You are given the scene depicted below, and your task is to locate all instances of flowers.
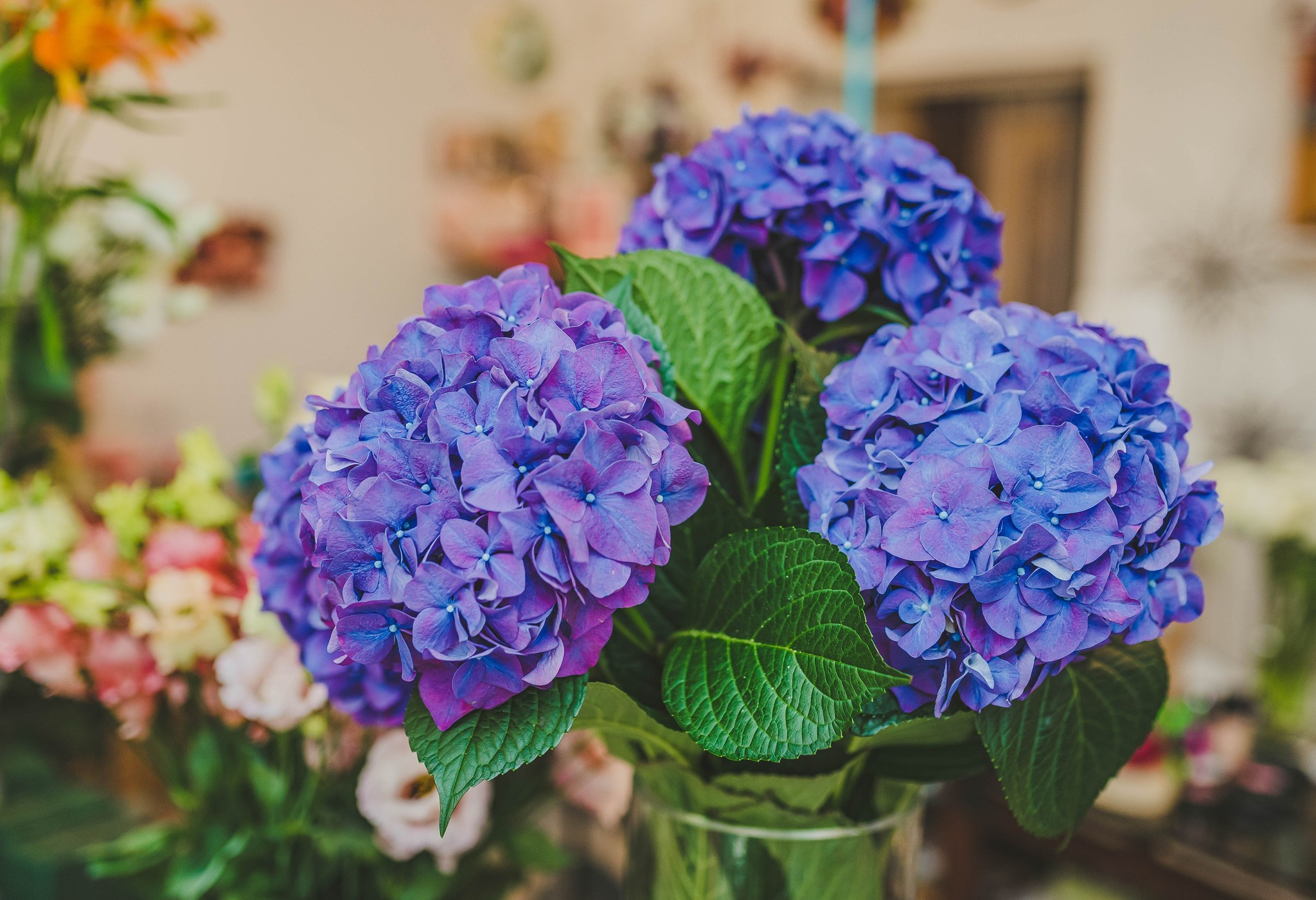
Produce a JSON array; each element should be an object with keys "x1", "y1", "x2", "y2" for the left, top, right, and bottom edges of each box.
[{"x1": 0, "y1": 0, "x2": 1265, "y2": 900}]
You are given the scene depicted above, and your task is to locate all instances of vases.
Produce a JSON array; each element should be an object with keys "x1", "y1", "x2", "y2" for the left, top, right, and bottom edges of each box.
[{"x1": 618, "y1": 759, "x2": 927, "y2": 900}]
